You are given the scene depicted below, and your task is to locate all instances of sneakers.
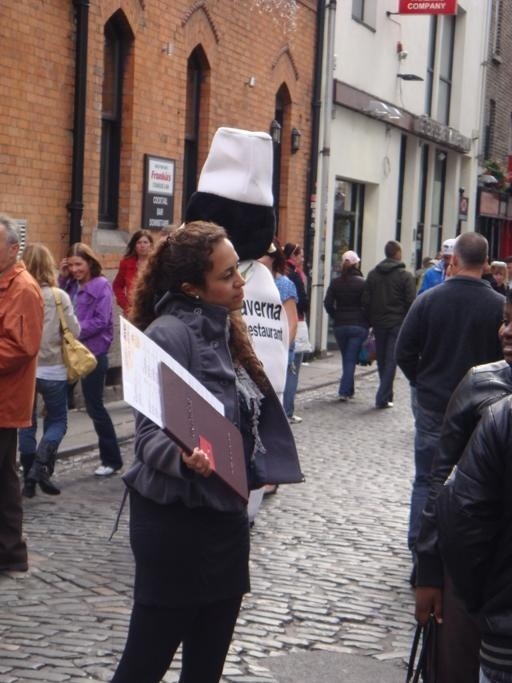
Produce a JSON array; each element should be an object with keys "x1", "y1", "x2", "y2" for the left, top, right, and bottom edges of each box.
[
  {"x1": 288, "y1": 414, "x2": 303, "y2": 423},
  {"x1": 377, "y1": 402, "x2": 394, "y2": 408},
  {"x1": 93, "y1": 463, "x2": 117, "y2": 476},
  {"x1": 340, "y1": 391, "x2": 358, "y2": 401}
]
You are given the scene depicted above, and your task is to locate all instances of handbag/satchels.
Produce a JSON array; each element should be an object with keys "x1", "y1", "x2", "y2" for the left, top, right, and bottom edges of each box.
[
  {"x1": 293, "y1": 320, "x2": 312, "y2": 353},
  {"x1": 62, "y1": 333, "x2": 97, "y2": 386},
  {"x1": 358, "y1": 328, "x2": 377, "y2": 366}
]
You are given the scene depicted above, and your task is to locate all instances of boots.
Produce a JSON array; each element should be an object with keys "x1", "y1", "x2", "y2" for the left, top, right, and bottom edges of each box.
[{"x1": 20, "y1": 441, "x2": 60, "y2": 496}]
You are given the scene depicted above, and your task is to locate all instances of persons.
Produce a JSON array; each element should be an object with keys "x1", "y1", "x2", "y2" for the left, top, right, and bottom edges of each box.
[
  {"x1": 2, "y1": 219, "x2": 43, "y2": 570},
  {"x1": 366, "y1": 241, "x2": 416, "y2": 409},
  {"x1": 110, "y1": 220, "x2": 306, "y2": 682},
  {"x1": 113, "y1": 230, "x2": 153, "y2": 316},
  {"x1": 260, "y1": 236, "x2": 308, "y2": 495},
  {"x1": 323, "y1": 250, "x2": 369, "y2": 402},
  {"x1": 394, "y1": 230, "x2": 512, "y2": 682},
  {"x1": 21, "y1": 241, "x2": 124, "y2": 496}
]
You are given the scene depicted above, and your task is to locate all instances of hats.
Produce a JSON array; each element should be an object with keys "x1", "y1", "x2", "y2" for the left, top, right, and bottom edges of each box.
[
  {"x1": 441, "y1": 238, "x2": 457, "y2": 256},
  {"x1": 342, "y1": 250, "x2": 360, "y2": 265}
]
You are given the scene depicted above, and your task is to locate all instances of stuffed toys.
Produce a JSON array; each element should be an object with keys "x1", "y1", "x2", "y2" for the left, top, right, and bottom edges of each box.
[{"x1": 186, "y1": 126, "x2": 288, "y2": 530}]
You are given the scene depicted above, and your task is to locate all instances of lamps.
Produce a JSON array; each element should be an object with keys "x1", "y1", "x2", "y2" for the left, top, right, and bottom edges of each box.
[
  {"x1": 245, "y1": 76, "x2": 256, "y2": 87},
  {"x1": 291, "y1": 128, "x2": 301, "y2": 153},
  {"x1": 162, "y1": 41, "x2": 174, "y2": 55},
  {"x1": 271, "y1": 119, "x2": 282, "y2": 144}
]
